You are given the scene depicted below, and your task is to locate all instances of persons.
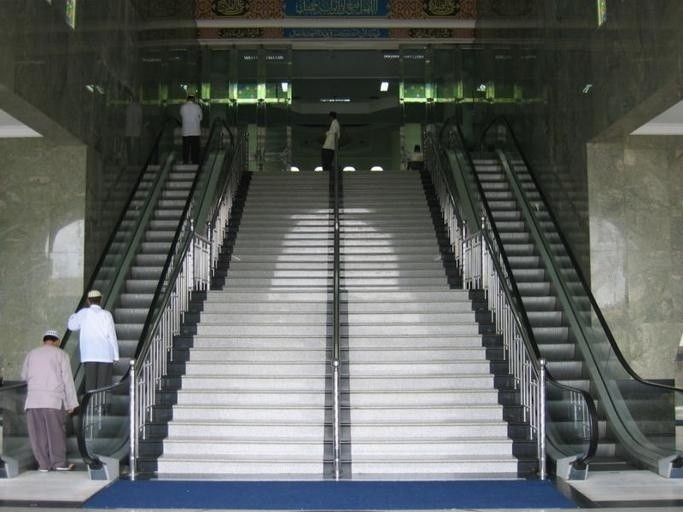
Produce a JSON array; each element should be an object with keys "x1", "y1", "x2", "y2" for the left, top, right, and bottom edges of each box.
[
  {"x1": 67, "y1": 289, "x2": 119, "y2": 406},
  {"x1": 123, "y1": 96, "x2": 144, "y2": 166},
  {"x1": 320, "y1": 111, "x2": 341, "y2": 171},
  {"x1": 178, "y1": 95, "x2": 203, "y2": 163},
  {"x1": 20, "y1": 330, "x2": 79, "y2": 472}
]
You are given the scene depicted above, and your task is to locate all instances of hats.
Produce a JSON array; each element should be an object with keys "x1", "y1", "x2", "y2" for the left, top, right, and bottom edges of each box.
[
  {"x1": 87, "y1": 289, "x2": 103, "y2": 298},
  {"x1": 41, "y1": 329, "x2": 61, "y2": 343}
]
